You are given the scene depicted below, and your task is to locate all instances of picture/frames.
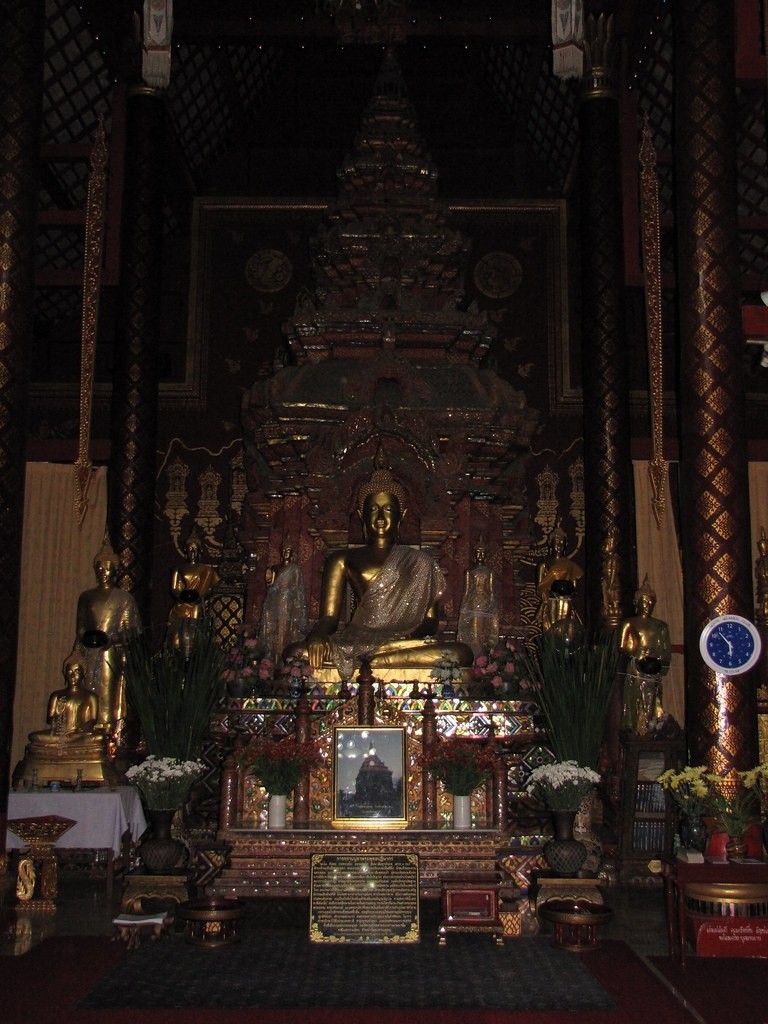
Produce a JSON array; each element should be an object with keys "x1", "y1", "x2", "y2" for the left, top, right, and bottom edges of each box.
[{"x1": 332, "y1": 724, "x2": 410, "y2": 823}]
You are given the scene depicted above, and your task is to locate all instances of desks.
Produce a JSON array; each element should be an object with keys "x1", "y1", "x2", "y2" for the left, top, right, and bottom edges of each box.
[{"x1": 7, "y1": 786, "x2": 149, "y2": 898}]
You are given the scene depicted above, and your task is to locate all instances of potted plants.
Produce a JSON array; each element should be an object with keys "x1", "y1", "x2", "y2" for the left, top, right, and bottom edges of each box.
[
  {"x1": 104, "y1": 608, "x2": 256, "y2": 847},
  {"x1": 516, "y1": 621, "x2": 624, "y2": 834}
]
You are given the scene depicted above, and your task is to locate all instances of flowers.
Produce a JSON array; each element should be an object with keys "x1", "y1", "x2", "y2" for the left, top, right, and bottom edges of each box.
[
  {"x1": 235, "y1": 733, "x2": 327, "y2": 796},
  {"x1": 430, "y1": 649, "x2": 464, "y2": 686},
  {"x1": 748, "y1": 753, "x2": 768, "y2": 826},
  {"x1": 213, "y1": 622, "x2": 270, "y2": 687},
  {"x1": 705, "y1": 768, "x2": 758, "y2": 837},
  {"x1": 656, "y1": 765, "x2": 724, "y2": 823},
  {"x1": 468, "y1": 636, "x2": 545, "y2": 692},
  {"x1": 522, "y1": 757, "x2": 603, "y2": 810},
  {"x1": 275, "y1": 646, "x2": 316, "y2": 676},
  {"x1": 123, "y1": 755, "x2": 208, "y2": 812},
  {"x1": 415, "y1": 735, "x2": 501, "y2": 796}
]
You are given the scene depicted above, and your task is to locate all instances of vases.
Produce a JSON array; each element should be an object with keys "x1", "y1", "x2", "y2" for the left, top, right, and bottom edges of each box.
[
  {"x1": 681, "y1": 816, "x2": 707, "y2": 854},
  {"x1": 705, "y1": 817, "x2": 732, "y2": 860},
  {"x1": 738, "y1": 817, "x2": 763, "y2": 858},
  {"x1": 288, "y1": 676, "x2": 307, "y2": 700},
  {"x1": 488, "y1": 677, "x2": 522, "y2": 703},
  {"x1": 441, "y1": 680, "x2": 456, "y2": 698},
  {"x1": 452, "y1": 794, "x2": 472, "y2": 832},
  {"x1": 268, "y1": 794, "x2": 286, "y2": 832},
  {"x1": 552, "y1": 808, "x2": 577, "y2": 842},
  {"x1": 140, "y1": 810, "x2": 186, "y2": 873},
  {"x1": 225, "y1": 669, "x2": 253, "y2": 699}
]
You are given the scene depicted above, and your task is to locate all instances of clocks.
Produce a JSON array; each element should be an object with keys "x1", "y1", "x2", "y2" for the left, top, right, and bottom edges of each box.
[{"x1": 699, "y1": 614, "x2": 761, "y2": 675}]
